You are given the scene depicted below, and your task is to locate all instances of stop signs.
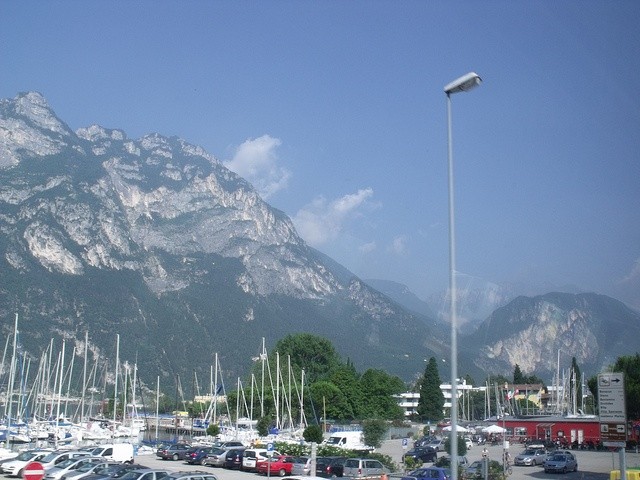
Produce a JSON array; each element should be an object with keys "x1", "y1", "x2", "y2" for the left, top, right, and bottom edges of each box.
[{"x1": 22, "y1": 461, "x2": 44, "y2": 480}]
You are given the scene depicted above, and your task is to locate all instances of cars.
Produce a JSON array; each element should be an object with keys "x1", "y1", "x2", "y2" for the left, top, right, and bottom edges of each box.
[
  {"x1": 514, "y1": 445, "x2": 547, "y2": 466},
  {"x1": 1, "y1": 451, "x2": 52, "y2": 478},
  {"x1": 545, "y1": 454, "x2": 576, "y2": 474},
  {"x1": 61, "y1": 461, "x2": 122, "y2": 480},
  {"x1": 77, "y1": 465, "x2": 151, "y2": 480},
  {"x1": 402, "y1": 447, "x2": 437, "y2": 462},
  {"x1": 43, "y1": 456, "x2": 107, "y2": 480},
  {"x1": 426, "y1": 440, "x2": 446, "y2": 452},
  {"x1": 120, "y1": 469, "x2": 172, "y2": 480},
  {"x1": 206, "y1": 448, "x2": 229, "y2": 467},
  {"x1": 256, "y1": 456, "x2": 295, "y2": 476},
  {"x1": 291, "y1": 456, "x2": 323, "y2": 475}
]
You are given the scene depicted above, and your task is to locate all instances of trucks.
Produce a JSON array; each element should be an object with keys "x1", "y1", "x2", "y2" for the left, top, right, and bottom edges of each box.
[
  {"x1": 326, "y1": 432, "x2": 376, "y2": 454},
  {"x1": 92, "y1": 444, "x2": 134, "y2": 465}
]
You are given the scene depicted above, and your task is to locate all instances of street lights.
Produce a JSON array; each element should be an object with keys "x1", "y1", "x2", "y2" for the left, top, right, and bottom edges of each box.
[{"x1": 444, "y1": 71, "x2": 483, "y2": 480}]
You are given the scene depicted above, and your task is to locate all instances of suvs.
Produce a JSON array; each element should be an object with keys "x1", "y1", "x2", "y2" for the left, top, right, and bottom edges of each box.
[
  {"x1": 463, "y1": 438, "x2": 473, "y2": 450},
  {"x1": 401, "y1": 467, "x2": 450, "y2": 480},
  {"x1": 159, "y1": 470, "x2": 217, "y2": 480},
  {"x1": 157, "y1": 444, "x2": 192, "y2": 461},
  {"x1": 41, "y1": 450, "x2": 92, "y2": 470},
  {"x1": 213, "y1": 441, "x2": 246, "y2": 452},
  {"x1": 316, "y1": 457, "x2": 348, "y2": 478},
  {"x1": 185, "y1": 446, "x2": 212, "y2": 465},
  {"x1": 226, "y1": 449, "x2": 242, "y2": 469}
]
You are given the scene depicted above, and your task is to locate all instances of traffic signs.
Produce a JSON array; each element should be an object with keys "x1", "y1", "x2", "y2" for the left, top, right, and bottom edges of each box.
[
  {"x1": 596, "y1": 373, "x2": 626, "y2": 441},
  {"x1": 267, "y1": 443, "x2": 274, "y2": 451}
]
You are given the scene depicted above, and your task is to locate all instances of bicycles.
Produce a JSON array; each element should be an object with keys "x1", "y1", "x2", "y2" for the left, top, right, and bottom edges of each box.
[{"x1": 506, "y1": 462, "x2": 513, "y2": 474}]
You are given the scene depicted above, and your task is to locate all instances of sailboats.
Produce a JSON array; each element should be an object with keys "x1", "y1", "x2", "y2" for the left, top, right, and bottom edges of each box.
[
  {"x1": 0, "y1": 311, "x2": 148, "y2": 443},
  {"x1": 169, "y1": 336, "x2": 330, "y2": 441}
]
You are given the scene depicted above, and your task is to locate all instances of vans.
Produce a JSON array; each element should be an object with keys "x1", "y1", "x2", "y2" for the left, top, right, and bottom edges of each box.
[
  {"x1": 242, "y1": 448, "x2": 282, "y2": 472},
  {"x1": 343, "y1": 458, "x2": 390, "y2": 480}
]
